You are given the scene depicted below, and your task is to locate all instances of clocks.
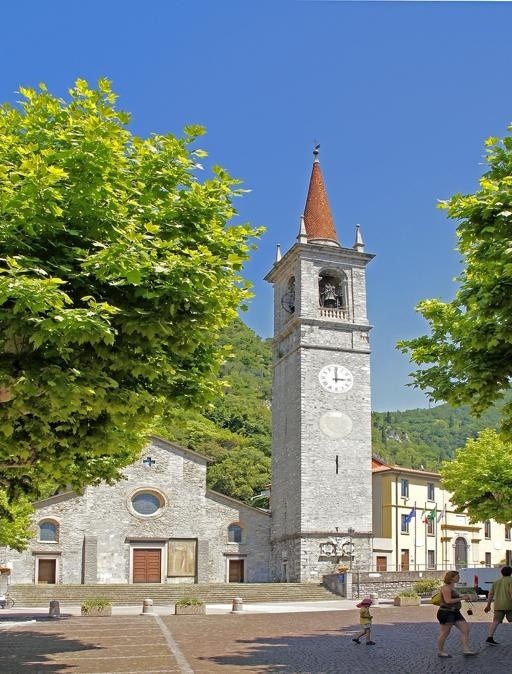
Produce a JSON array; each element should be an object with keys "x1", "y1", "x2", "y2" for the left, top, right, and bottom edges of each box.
[{"x1": 317, "y1": 362, "x2": 354, "y2": 395}]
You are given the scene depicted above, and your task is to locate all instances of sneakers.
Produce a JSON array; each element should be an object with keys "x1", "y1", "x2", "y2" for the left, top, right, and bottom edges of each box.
[
  {"x1": 352, "y1": 638, "x2": 377, "y2": 646},
  {"x1": 437, "y1": 637, "x2": 500, "y2": 658}
]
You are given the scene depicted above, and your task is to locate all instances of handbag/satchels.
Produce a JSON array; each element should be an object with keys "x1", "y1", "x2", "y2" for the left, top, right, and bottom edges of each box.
[{"x1": 430, "y1": 591, "x2": 441, "y2": 606}]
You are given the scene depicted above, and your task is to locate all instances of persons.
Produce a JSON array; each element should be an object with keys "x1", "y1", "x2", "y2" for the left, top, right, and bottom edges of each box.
[
  {"x1": 351, "y1": 598, "x2": 376, "y2": 645},
  {"x1": 437, "y1": 571, "x2": 478, "y2": 657},
  {"x1": 483, "y1": 566, "x2": 511, "y2": 644}
]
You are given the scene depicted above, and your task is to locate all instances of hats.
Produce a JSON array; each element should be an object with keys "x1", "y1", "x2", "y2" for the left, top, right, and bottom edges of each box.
[{"x1": 355, "y1": 598, "x2": 372, "y2": 608}]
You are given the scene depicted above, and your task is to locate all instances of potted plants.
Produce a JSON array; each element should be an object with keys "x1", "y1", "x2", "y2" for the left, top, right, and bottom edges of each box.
[
  {"x1": 394, "y1": 590, "x2": 421, "y2": 605},
  {"x1": 82, "y1": 597, "x2": 112, "y2": 616},
  {"x1": 175, "y1": 597, "x2": 208, "y2": 615}
]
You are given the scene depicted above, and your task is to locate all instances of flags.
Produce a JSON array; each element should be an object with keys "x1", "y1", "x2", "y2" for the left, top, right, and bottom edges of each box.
[
  {"x1": 420, "y1": 508, "x2": 429, "y2": 524},
  {"x1": 404, "y1": 505, "x2": 416, "y2": 523},
  {"x1": 437, "y1": 507, "x2": 445, "y2": 523},
  {"x1": 427, "y1": 507, "x2": 436, "y2": 522}
]
